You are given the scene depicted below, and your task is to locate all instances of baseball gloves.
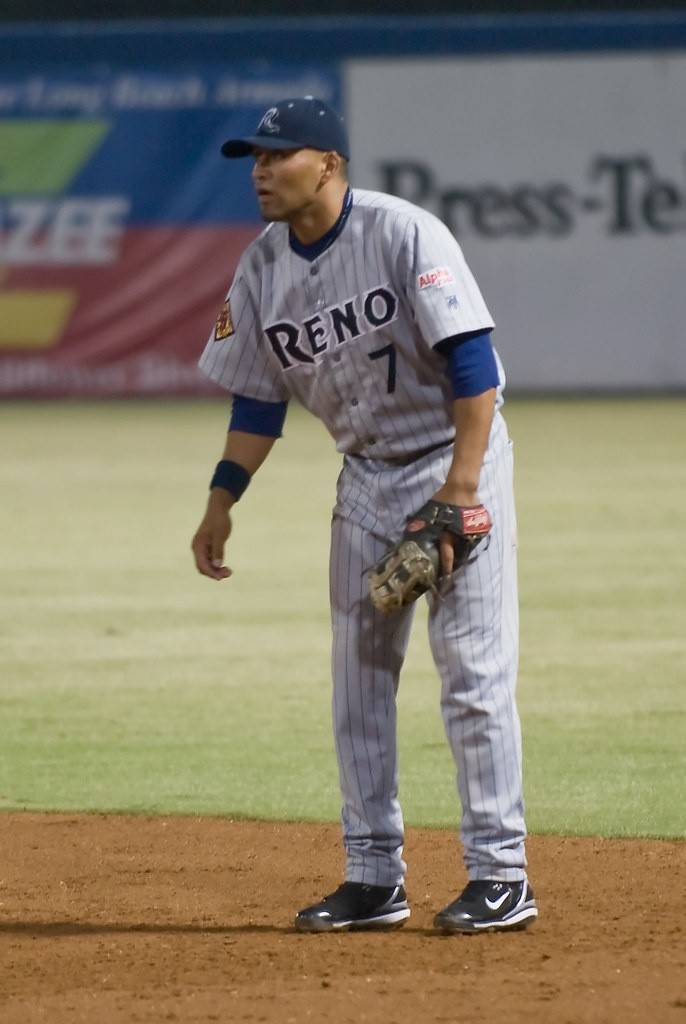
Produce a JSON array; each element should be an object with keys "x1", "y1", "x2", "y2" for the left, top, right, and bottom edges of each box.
[{"x1": 360, "y1": 499, "x2": 494, "y2": 639}]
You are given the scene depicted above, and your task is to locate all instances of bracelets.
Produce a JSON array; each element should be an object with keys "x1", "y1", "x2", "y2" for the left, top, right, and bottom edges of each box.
[{"x1": 209, "y1": 459, "x2": 251, "y2": 501}]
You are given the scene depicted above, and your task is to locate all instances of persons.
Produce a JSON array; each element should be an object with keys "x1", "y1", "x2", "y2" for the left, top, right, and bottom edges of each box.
[{"x1": 191, "y1": 96, "x2": 538, "y2": 934}]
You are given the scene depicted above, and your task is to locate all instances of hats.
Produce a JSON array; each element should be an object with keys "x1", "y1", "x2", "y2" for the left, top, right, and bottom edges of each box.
[{"x1": 221, "y1": 94, "x2": 349, "y2": 160}]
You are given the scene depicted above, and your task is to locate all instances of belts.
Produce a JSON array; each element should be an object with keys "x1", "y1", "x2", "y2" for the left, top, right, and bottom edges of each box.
[{"x1": 382, "y1": 437, "x2": 454, "y2": 467}]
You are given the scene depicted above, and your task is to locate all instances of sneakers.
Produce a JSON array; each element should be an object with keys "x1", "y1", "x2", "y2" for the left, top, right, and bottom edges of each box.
[
  {"x1": 295, "y1": 881, "x2": 410, "y2": 933},
  {"x1": 433, "y1": 880, "x2": 538, "y2": 935}
]
